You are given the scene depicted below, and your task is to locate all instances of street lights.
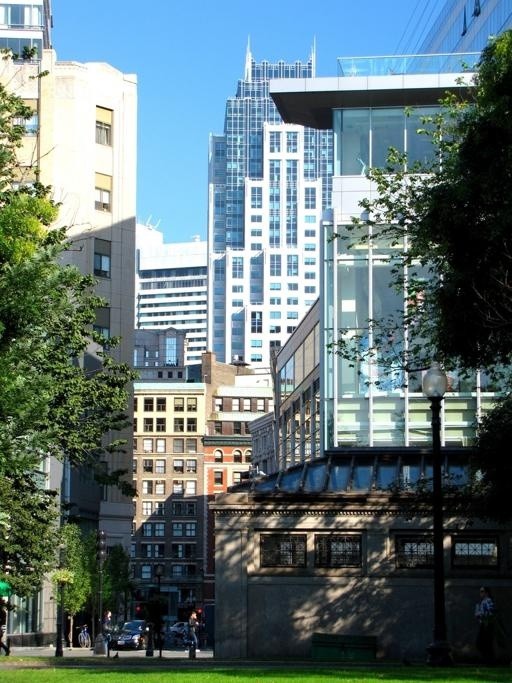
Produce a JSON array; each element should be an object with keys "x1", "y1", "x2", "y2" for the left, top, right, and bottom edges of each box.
[{"x1": 421, "y1": 362, "x2": 448, "y2": 668}]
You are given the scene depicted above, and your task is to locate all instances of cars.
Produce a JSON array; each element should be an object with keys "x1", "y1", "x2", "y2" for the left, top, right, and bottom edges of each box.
[{"x1": 111, "y1": 620, "x2": 189, "y2": 650}]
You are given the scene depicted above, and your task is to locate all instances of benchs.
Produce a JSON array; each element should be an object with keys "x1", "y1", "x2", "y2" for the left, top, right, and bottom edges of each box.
[{"x1": 309, "y1": 632, "x2": 379, "y2": 659}]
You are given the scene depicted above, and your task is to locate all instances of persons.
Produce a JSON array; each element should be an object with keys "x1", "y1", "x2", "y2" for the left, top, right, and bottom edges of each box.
[
  {"x1": 0, "y1": 607, "x2": 11, "y2": 656},
  {"x1": 185, "y1": 611, "x2": 201, "y2": 652},
  {"x1": 102, "y1": 608, "x2": 112, "y2": 650},
  {"x1": 474, "y1": 586, "x2": 497, "y2": 667}
]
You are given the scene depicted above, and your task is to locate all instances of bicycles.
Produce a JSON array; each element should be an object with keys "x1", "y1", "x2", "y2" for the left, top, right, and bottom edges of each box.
[{"x1": 76, "y1": 624, "x2": 91, "y2": 648}]
